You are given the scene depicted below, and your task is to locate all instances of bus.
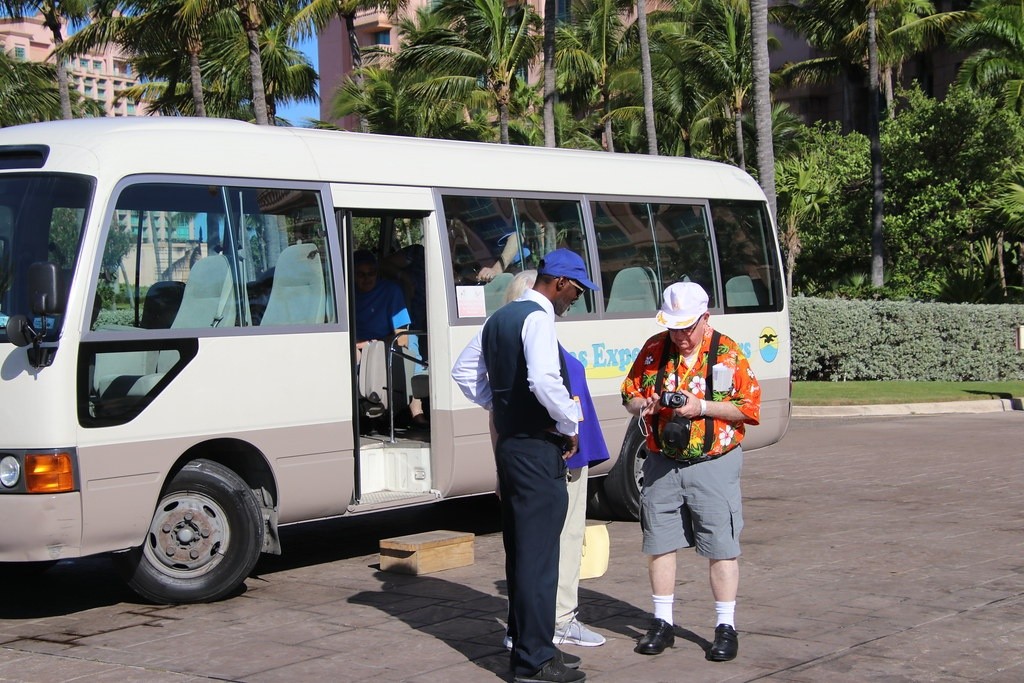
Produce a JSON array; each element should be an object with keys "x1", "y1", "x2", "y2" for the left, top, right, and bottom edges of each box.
[{"x1": 0, "y1": 117, "x2": 789, "y2": 604}]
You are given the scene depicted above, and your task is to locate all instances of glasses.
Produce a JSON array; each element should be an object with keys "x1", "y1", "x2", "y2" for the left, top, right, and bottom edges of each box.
[
  {"x1": 356, "y1": 270, "x2": 377, "y2": 277},
  {"x1": 554, "y1": 276, "x2": 585, "y2": 296},
  {"x1": 667, "y1": 313, "x2": 706, "y2": 334}
]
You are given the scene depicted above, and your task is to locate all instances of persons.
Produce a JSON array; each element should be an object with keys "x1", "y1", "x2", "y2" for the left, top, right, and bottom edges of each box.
[
  {"x1": 451, "y1": 270, "x2": 611, "y2": 647},
  {"x1": 355, "y1": 247, "x2": 411, "y2": 434},
  {"x1": 466, "y1": 199, "x2": 535, "y2": 283},
  {"x1": 620, "y1": 282, "x2": 761, "y2": 661}
]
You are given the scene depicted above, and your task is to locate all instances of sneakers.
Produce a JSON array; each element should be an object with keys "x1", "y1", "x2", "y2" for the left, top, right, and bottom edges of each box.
[
  {"x1": 553, "y1": 611, "x2": 605, "y2": 646},
  {"x1": 516, "y1": 657, "x2": 586, "y2": 683},
  {"x1": 554, "y1": 648, "x2": 582, "y2": 669}
]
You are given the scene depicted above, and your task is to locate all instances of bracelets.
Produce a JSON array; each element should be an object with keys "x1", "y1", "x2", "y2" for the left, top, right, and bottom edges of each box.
[
  {"x1": 368, "y1": 338, "x2": 373, "y2": 344},
  {"x1": 699, "y1": 399, "x2": 706, "y2": 415}
]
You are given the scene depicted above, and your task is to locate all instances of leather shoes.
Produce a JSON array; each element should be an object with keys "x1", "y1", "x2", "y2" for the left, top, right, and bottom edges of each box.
[
  {"x1": 710, "y1": 624, "x2": 738, "y2": 659},
  {"x1": 639, "y1": 617, "x2": 675, "y2": 653}
]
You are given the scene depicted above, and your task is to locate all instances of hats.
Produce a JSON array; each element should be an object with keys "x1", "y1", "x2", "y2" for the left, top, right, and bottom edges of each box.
[
  {"x1": 656, "y1": 281, "x2": 709, "y2": 329},
  {"x1": 538, "y1": 248, "x2": 598, "y2": 291}
]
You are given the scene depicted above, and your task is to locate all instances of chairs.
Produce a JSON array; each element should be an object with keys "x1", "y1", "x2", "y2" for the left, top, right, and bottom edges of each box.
[
  {"x1": 141, "y1": 244, "x2": 327, "y2": 329},
  {"x1": 479, "y1": 268, "x2": 764, "y2": 308}
]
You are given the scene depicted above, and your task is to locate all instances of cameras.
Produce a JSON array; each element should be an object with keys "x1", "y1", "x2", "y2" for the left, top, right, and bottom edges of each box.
[{"x1": 660, "y1": 391, "x2": 687, "y2": 408}]
